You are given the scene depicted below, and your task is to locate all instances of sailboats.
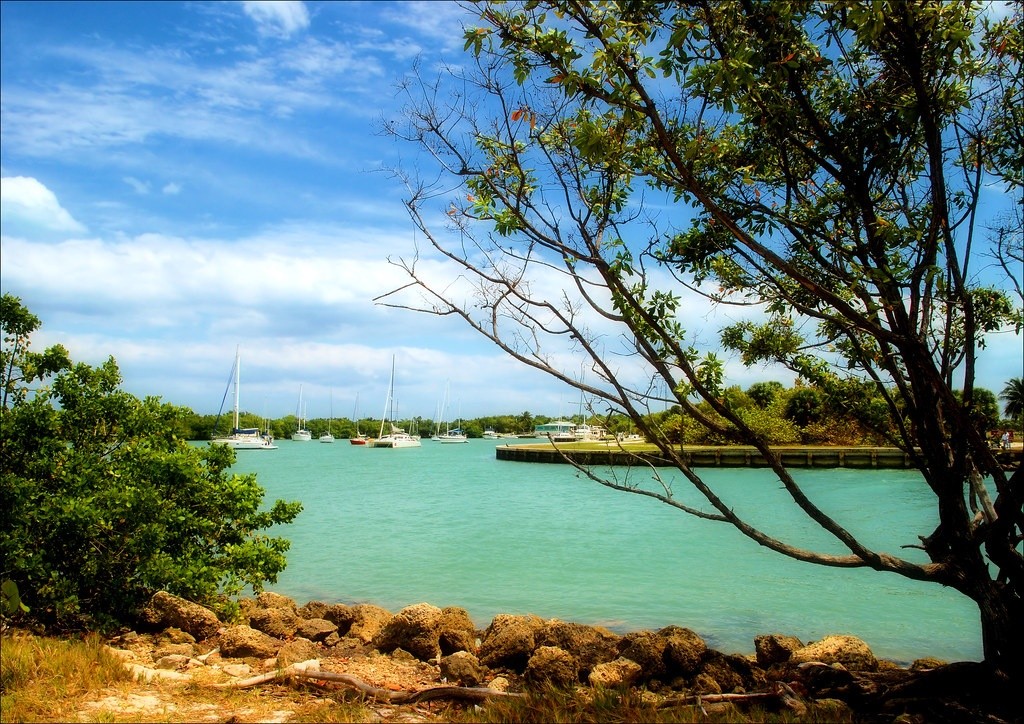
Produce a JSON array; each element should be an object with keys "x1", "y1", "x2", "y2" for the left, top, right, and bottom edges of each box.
[
  {"x1": 319, "y1": 388, "x2": 335, "y2": 442},
  {"x1": 373, "y1": 353, "x2": 422, "y2": 449},
  {"x1": 210, "y1": 344, "x2": 277, "y2": 449},
  {"x1": 429, "y1": 378, "x2": 468, "y2": 442},
  {"x1": 554, "y1": 369, "x2": 576, "y2": 442},
  {"x1": 349, "y1": 391, "x2": 370, "y2": 444},
  {"x1": 292, "y1": 384, "x2": 312, "y2": 441}
]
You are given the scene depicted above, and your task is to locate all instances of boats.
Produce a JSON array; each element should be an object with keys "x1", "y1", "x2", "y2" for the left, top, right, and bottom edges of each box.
[{"x1": 482, "y1": 432, "x2": 498, "y2": 439}]
